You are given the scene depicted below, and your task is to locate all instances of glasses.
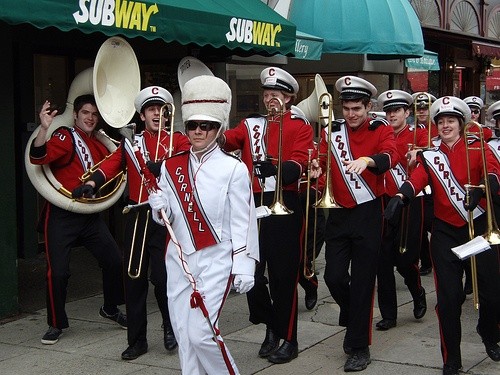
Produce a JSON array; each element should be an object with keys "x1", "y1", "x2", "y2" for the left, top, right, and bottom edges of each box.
[{"x1": 186, "y1": 121, "x2": 215, "y2": 131}]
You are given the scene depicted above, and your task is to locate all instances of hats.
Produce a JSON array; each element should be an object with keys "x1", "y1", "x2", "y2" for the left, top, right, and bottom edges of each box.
[
  {"x1": 411, "y1": 92, "x2": 437, "y2": 108},
  {"x1": 430, "y1": 96, "x2": 471, "y2": 127},
  {"x1": 378, "y1": 90, "x2": 413, "y2": 112},
  {"x1": 335, "y1": 76, "x2": 377, "y2": 100},
  {"x1": 260, "y1": 67, "x2": 299, "y2": 95},
  {"x1": 134, "y1": 86, "x2": 174, "y2": 114},
  {"x1": 463, "y1": 96, "x2": 483, "y2": 109},
  {"x1": 488, "y1": 101, "x2": 500, "y2": 118},
  {"x1": 180, "y1": 75, "x2": 231, "y2": 132}
]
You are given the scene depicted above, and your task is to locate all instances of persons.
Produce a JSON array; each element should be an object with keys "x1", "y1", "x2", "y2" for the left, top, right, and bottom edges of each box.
[
  {"x1": 83, "y1": 86, "x2": 192, "y2": 359},
  {"x1": 462, "y1": 96, "x2": 493, "y2": 295},
  {"x1": 310, "y1": 76, "x2": 402, "y2": 372},
  {"x1": 410, "y1": 92, "x2": 439, "y2": 275},
  {"x1": 486, "y1": 99, "x2": 500, "y2": 181},
  {"x1": 367, "y1": 111, "x2": 389, "y2": 121},
  {"x1": 148, "y1": 75, "x2": 260, "y2": 375},
  {"x1": 217, "y1": 67, "x2": 315, "y2": 364},
  {"x1": 376, "y1": 90, "x2": 430, "y2": 330},
  {"x1": 383, "y1": 96, "x2": 500, "y2": 375},
  {"x1": 29, "y1": 93, "x2": 128, "y2": 345}
]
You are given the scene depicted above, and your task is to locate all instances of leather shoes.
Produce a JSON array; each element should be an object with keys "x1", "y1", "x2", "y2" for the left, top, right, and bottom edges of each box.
[
  {"x1": 376, "y1": 319, "x2": 396, "y2": 330},
  {"x1": 259, "y1": 329, "x2": 280, "y2": 356},
  {"x1": 161, "y1": 320, "x2": 177, "y2": 350},
  {"x1": 413, "y1": 287, "x2": 427, "y2": 319},
  {"x1": 482, "y1": 338, "x2": 500, "y2": 360},
  {"x1": 305, "y1": 288, "x2": 317, "y2": 309},
  {"x1": 268, "y1": 341, "x2": 298, "y2": 363},
  {"x1": 122, "y1": 339, "x2": 147, "y2": 359},
  {"x1": 443, "y1": 366, "x2": 460, "y2": 375}
]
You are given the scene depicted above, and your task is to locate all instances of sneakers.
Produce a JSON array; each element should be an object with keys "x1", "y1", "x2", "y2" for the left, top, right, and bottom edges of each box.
[
  {"x1": 343, "y1": 331, "x2": 353, "y2": 355},
  {"x1": 99, "y1": 305, "x2": 128, "y2": 329},
  {"x1": 41, "y1": 326, "x2": 63, "y2": 344},
  {"x1": 344, "y1": 346, "x2": 371, "y2": 371}
]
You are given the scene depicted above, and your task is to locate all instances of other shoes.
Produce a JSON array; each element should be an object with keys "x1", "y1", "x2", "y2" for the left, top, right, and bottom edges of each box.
[{"x1": 419, "y1": 266, "x2": 432, "y2": 275}]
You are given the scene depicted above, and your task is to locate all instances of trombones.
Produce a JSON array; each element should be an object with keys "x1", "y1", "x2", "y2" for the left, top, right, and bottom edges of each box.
[
  {"x1": 393, "y1": 92, "x2": 431, "y2": 255},
  {"x1": 464, "y1": 121, "x2": 500, "y2": 310},
  {"x1": 126, "y1": 102, "x2": 175, "y2": 279},
  {"x1": 304, "y1": 92, "x2": 344, "y2": 277},
  {"x1": 251, "y1": 97, "x2": 295, "y2": 234}
]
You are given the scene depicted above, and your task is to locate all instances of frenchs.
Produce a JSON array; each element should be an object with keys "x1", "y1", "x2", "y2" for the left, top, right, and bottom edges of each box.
[
  {"x1": 178, "y1": 56, "x2": 215, "y2": 131},
  {"x1": 25, "y1": 36, "x2": 141, "y2": 213},
  {"x1": 295, "y1": 73, "x2": 335, "y2": 126}
]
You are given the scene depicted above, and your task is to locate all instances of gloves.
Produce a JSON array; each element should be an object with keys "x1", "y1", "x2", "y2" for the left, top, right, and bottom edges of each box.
[
  {"x1": 72, "y1": 185, "x2": 94, "y2": 198},
  {"x1": 148, "y1": 190, "x2": 166, "y2": 212},
  {"x1": 383, "y1": 195, "x2": 404, "y2": 220},
  {"x1": 234, "y1": 276, "x2": 255, "y2": 294},
  {"x1": 253, "y1": 155, "x2": 277, "y2": 179},
  {"x1": 463, "y1": 188, "x2": 484, "y2": 210},
  {"x1": 280, "y1": 160, "x2": 300, "y2": 186},
  {"x1": 146, "y1": 161, "x2": 162, "y2": 178}
]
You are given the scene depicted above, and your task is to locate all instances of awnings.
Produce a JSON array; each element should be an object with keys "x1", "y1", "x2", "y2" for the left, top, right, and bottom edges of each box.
[
  {"x1": 0, "y1": 0, "x2": 440, "y2": 75},
  {"x1": 420, "y1": 23, "x2": 500, "y2": 92}
]
